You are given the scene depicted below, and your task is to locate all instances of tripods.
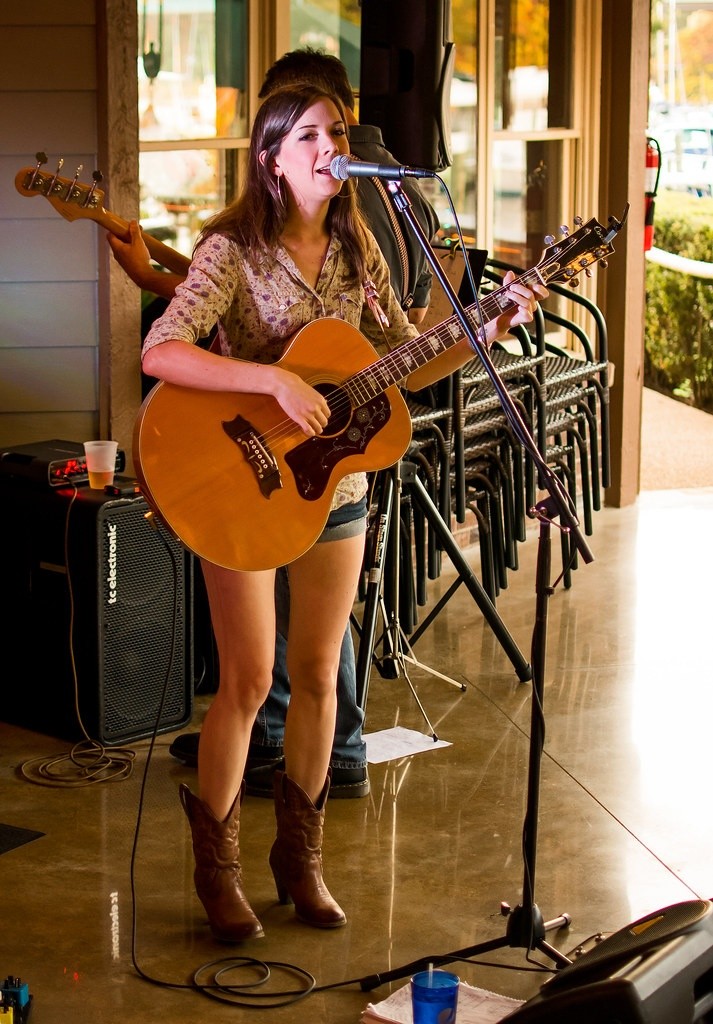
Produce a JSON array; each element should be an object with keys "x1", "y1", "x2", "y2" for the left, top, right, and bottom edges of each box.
[{"x1": 360, "y1": 175, "x2": 591, "y2": 995}]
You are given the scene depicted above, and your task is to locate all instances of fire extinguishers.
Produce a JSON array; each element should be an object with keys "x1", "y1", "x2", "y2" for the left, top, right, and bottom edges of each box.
[{"x1": 645, "y1": 137, "x2": 661, "y2": 252}]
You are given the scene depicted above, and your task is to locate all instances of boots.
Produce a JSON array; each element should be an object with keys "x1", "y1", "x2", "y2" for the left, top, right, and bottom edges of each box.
[
  {"x1": 270, "y1": 770, "x2": 349, "y2": 928},
  {"x1": 177, "y1": 781, "x2": 265, "y2": 942}
]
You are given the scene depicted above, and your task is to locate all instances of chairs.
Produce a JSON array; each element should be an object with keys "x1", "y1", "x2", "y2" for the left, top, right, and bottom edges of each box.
[{"x1": 357, "y1": 259, "x2": 610, "y2": 632}]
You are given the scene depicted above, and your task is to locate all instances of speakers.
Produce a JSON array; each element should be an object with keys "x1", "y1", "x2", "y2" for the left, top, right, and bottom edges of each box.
[
  {"x1": 0, "y1": 472, "x2": 195, "y2": 747},
  {"x1": 359, "y1": 0, "x2": 456, "y2": 172},
  {"x1": 494, "y1": 899, "x2": 713, "y2": 1024}
]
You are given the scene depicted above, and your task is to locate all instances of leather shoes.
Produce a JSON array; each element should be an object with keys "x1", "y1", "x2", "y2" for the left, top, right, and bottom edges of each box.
[{"x1": 165, "y1": 718, "x2": 370, "y2": 800}]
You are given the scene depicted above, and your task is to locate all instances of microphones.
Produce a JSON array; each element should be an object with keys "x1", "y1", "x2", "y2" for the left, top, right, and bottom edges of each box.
[{"x1": 329, "y1": 154, "x2": 435, "y2": 180}]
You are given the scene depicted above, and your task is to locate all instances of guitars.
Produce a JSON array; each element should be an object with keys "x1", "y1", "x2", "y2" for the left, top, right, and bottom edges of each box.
[
  {"x1": 132, "y1": 201, "x2": 632, "y2": 573},
  {"x1": 14, "y1": 150, "x2": 191, "y2": 275}
]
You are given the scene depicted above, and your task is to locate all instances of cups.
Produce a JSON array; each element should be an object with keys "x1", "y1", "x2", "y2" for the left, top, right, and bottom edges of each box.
[
  {"x1": 84, "y1": 441, "x2": 118, "y2": 489},
  {"x1": 410, "y1": 971, "x2": 460, "y2": 1024}
]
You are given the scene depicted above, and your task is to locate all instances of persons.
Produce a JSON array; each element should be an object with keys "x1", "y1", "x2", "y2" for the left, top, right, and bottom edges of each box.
[
  {"x1": 107, "y1": 46, "x2": 439, "y2": 799},
  {"x1": 140, "y1": 82, "x2": 549, "y2": 946}
]
